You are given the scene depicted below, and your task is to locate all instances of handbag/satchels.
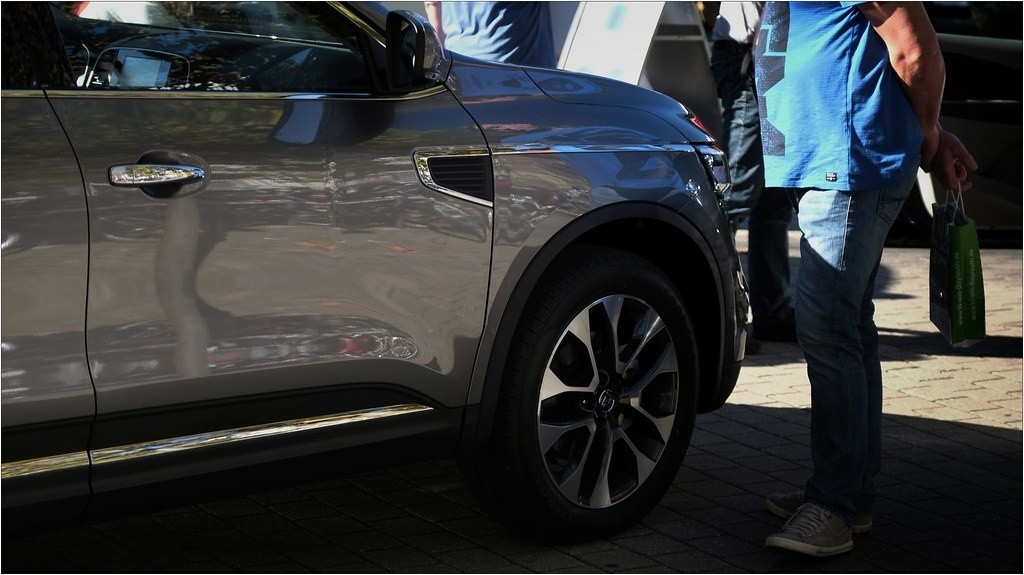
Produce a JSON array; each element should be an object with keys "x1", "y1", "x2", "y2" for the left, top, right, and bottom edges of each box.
[{"x1": 929, "y1": 180, "x2": 987, "y2": 351}]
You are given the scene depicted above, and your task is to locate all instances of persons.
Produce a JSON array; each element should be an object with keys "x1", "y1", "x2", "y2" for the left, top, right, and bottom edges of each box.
[
  {"x1": 752, "y1": 2, "x2": 978, "y2": 557},
  {"x1": 424, "y1": 1, "x2": 558, "y2": 70},
  {"x1": 710, "y1": 2, "x2": 799, "y2": 358}
]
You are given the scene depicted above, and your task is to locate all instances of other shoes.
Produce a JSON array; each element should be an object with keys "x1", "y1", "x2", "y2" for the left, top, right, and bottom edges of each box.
[{"x1": 753, "y1": 296, "x2": 799, "y2": 344}]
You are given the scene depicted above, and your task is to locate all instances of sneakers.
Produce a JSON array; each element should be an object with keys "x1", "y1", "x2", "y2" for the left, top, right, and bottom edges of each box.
[
  {"x1": 767, "y1": 492, "x2": 874, "y2": 533},
  {"x1": 766, "y1": 504, "x2": 853, "y2": 558}
]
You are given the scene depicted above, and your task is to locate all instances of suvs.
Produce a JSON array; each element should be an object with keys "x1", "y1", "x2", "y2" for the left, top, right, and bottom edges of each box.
[{"x1": 1, "y1": 1, "x2": 754, "y2": 544}]
